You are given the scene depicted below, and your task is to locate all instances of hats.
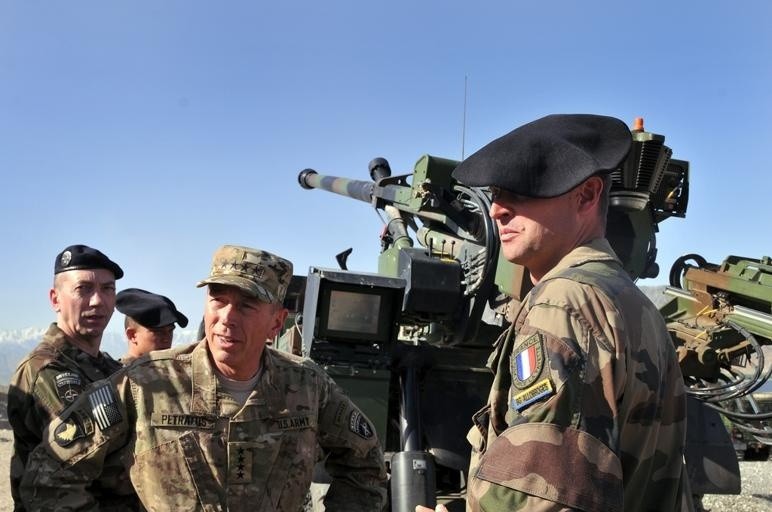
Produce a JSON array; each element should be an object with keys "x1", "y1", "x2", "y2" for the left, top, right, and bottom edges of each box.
[
  {"x1": 196, "y1": 244, "x2": 293, "y2": 304},
  {"x1": 54, "y1": 245, "x2": 124, "y2": 279},
  {"x1": 115, "y1": 289, "x2": 189, "y2": 328},
  {"x1": 450, "y1": 114, "x2": 632, "y2": 198}
]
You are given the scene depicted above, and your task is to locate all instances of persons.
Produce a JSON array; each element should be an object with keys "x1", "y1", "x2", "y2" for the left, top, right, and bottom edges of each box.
[
  {"x1": 115, "y1": 290, "x2": 188, "y2": 367},
  {"x1": 15, "y1": 246, "x2": 387, "y2": 512},
  {"x1": 453, "y1": 114, "x2": 687, "y2": 512},
  {"x1": 6, "y1": 244, "x2": 140, "y2": 511}
]
